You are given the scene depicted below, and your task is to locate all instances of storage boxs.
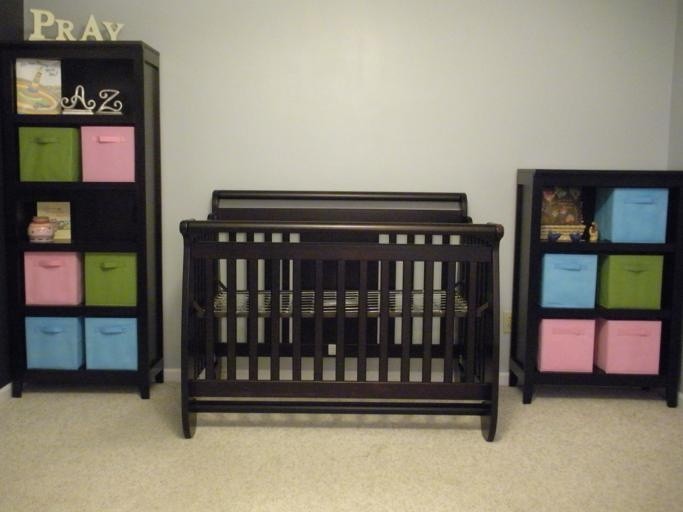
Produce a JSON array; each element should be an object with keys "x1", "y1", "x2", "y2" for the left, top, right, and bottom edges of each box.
[
  {"x1": 14, "y1": 122, "x2": 139, "y2": 184},
  {"x1": 536, "y1": 187, "x2": 669, "y2": 375},
  {"x1": 18, "y1": 247, "x2": 140, "y2": 374}
]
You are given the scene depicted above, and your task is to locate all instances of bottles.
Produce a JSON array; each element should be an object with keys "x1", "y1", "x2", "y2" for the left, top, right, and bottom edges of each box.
[{"x1": 26, "y1": 216, "x2": 55, "y2": 242}]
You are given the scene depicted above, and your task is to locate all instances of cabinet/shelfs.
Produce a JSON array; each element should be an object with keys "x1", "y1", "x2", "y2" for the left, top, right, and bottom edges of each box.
[
  {"x1": 2, "y1": 38, "x2": 166, "y2": 399},
  {"x1": 508, "y1": 167, "x2": 683, "y2": 408}
]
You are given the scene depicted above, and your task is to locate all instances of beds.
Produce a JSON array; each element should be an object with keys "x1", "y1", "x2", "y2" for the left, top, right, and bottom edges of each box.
[{"x1": 178, "y1": 188, "x2": 503, "y2": 443}]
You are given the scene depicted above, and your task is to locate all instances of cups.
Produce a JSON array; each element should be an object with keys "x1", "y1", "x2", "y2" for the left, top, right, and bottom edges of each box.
[{"x1": 547, "y1": 231, "x2": 581, "y2": 243}]
